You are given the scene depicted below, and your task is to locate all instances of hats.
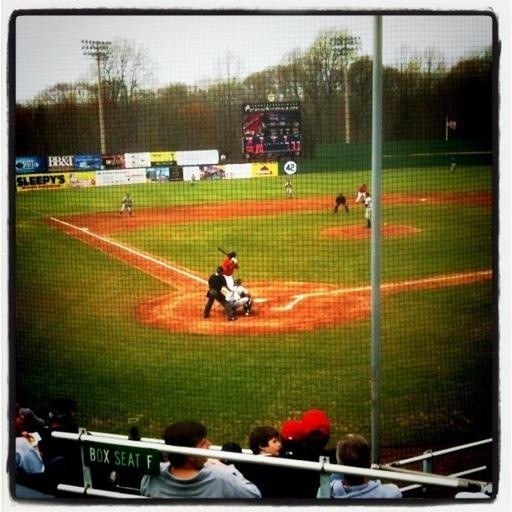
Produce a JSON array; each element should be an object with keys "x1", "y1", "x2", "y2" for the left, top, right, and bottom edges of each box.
[{"x1": 280, "y1": 408, "x2": 333, "y2": 444}]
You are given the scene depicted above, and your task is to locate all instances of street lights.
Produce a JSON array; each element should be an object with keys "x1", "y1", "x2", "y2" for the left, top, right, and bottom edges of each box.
[
  {"x1": 81, "y1": 40, "x2": 112, "y2": 156},
  {"x1": 330, "y1": 37, "x2": 362, "y2": 143}
]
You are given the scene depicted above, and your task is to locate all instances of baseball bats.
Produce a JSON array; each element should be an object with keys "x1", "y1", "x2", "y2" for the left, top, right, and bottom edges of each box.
[{"x1": 217, "y1": 248, "x2": 229, "y2": 256}]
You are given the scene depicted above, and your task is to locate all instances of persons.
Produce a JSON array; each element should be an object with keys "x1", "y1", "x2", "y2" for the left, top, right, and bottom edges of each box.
[
  {"x1": 119, "y1": 193, "x2": 133, "y2": 217},
  {"x1": 364, "y1": 193, "x2": 373, "y2": 228},
  {"x1": 203, "y1": 267, "x2": 238, "y2": 321},
  {"x1": 284, "y1": 179, "x2": 293, "y2": 199},
  {"x1": 220, "y1": 251, "x2": 239, "y2": 288},
  {"x1": 355, "y1": 183, "x2": 368, "y2": 204},
  {"x1": 333, "y1": 193, "x2": 350, "y2": 215},
  {"x1": 226, "y1": 279, "x2": 253, "y2": 316},
  {"x1": 449, "y1": 156, "x2": 457, "y2": 174}
]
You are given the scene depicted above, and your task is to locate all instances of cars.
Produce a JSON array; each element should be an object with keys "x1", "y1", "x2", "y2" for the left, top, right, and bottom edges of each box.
[{"x1": 192, "y1": 168, "x2": 224, "y2": 180}]
